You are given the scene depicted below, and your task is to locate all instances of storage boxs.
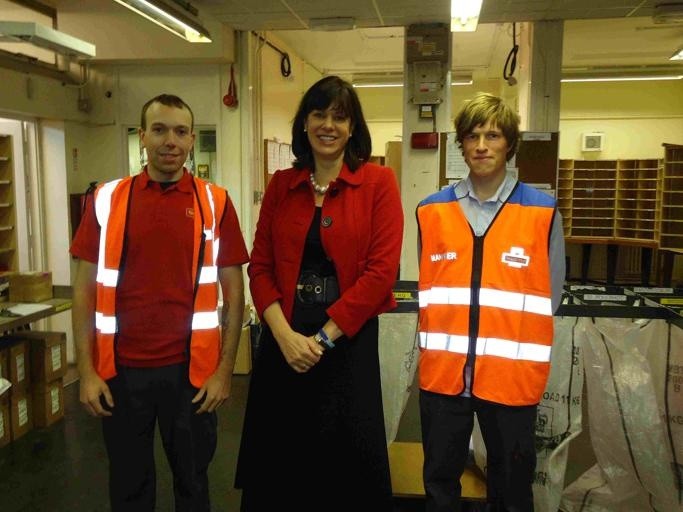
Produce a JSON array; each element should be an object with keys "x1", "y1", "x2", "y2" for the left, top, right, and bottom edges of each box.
[
  {"x1": 218, "y1": 318, "x2": 254, "y2": 375},
  {"x1": 8, "y1": 271, "x2": 53, "y2": 303},
  {"x1": 0, "y1": 329, "x2": 69, "y2": 448},
  {"x1": 217, "y1": 302, "x2": 252, "y2": 327}
]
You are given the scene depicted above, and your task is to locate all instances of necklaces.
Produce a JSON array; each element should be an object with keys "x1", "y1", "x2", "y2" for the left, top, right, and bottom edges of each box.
[{"x1": 309, "y1": 170, "x2": 336, "y2": 194}]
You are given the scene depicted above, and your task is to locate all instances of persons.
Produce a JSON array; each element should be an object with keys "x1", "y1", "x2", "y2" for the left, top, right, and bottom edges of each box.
[
  {"x1": 412, "y1": 90, "x2": 566, "y2": 512},
  {"x1": 69, "y1": 94, "x2": 250, "y2": 512},
  {"x1": 231, "y1": 75, "x2": 404, "y2": 512}
]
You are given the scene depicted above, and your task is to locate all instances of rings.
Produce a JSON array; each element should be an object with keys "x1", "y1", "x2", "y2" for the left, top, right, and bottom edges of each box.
[{"x1": 301, "y1": 361, "x2": 306, "y2": 370}]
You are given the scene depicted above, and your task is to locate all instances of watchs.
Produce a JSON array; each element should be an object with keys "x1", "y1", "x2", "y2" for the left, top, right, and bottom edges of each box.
[{"x1": 314, "y1": 332, "x2": 330, "y2": 350}]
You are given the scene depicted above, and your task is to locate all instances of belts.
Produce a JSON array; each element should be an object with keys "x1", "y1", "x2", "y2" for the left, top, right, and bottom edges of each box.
[{"x1": 294, "y1": 272, "x2": 339, "y2": 311}]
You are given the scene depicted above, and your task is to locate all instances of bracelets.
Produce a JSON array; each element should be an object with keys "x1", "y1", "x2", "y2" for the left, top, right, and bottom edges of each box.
[{"x1": 318, "y1": 328, "x2": 335, "y2": 349}]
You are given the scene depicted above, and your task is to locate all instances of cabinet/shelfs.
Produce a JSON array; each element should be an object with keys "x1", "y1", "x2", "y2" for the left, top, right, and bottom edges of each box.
[
  {"x1": 1, "y1": 133, "x2": 20, "y2": 306},
  {"x1": 558, "y1": 142, "x2": 683, "y2": 287}
]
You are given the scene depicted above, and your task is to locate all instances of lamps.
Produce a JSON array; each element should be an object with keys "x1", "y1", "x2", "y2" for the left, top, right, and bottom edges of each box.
[
  {"x1": 113, "y1": 0, "x2": 212, "y2": 44},
  {"x1": 450, "y1": 0, "x2": 484, "y2": 33}
]
